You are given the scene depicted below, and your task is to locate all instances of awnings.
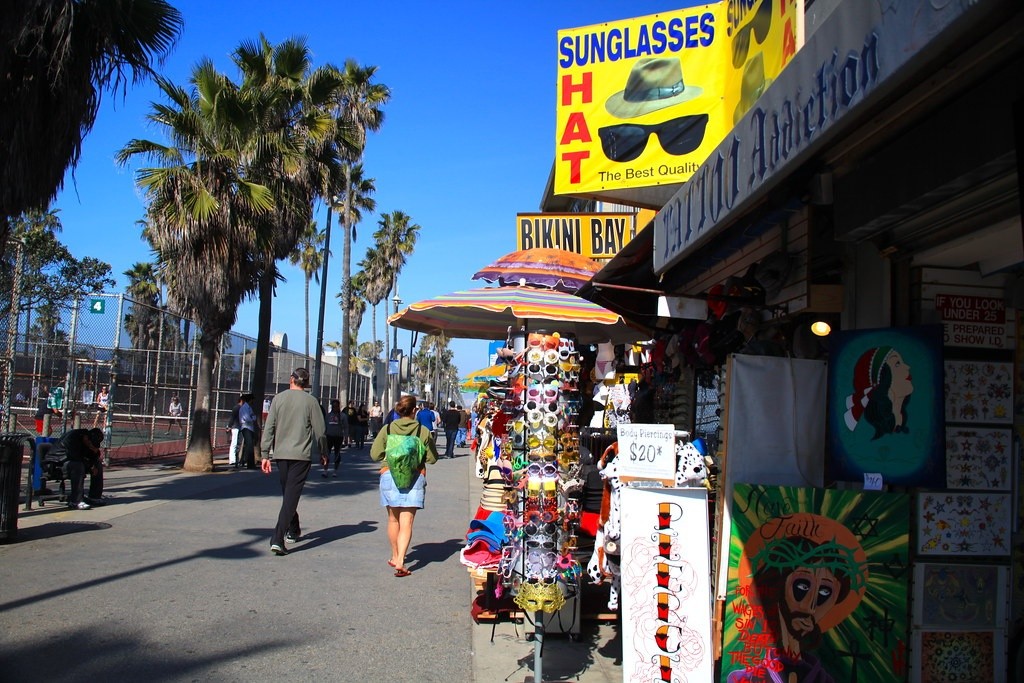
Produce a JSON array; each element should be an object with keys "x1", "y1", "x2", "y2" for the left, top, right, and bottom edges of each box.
[{"x1": 573, "y1": 219, "x2": 667, "y2": 304}]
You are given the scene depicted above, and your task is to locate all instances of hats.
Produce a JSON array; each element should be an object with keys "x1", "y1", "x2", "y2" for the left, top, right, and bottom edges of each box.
[
  {"x1": 86, "y1": 428, "x2": 104, "y2": 449},
  {"x1": 734, "y1": 52, "x2": 771, "y2": 126},
  {"x1": 469, "y1": 343, "x2": 524, "y2": 624},
  {"x1": 606, "y1": 58, "x2": 702, "y2": 118}
]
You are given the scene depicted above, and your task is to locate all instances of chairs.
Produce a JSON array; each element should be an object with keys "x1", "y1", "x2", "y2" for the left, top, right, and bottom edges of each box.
[{"x1": 37, "y1": 442, "x2": 71, "y2": 507}]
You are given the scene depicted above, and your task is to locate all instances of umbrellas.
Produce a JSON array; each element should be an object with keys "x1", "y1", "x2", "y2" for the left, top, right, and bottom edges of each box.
[
  {"x1": 470, "y1": 245, "x2": 606, "y2": 288},
  {"x1": 384, "y1": 287, "x2": 627, "y2": 340},
  {"x1": 457, "y1": 364, "x2": 512, "y2": 393}
]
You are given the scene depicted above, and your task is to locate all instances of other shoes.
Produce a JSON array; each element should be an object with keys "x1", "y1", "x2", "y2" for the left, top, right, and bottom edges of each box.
[
  {"x1": 332, "y1": 471, "x2": 338, "y2": 477},
  {"x1": 286, "y1": 528, "x2": 301, "y2": 544},
  {"x1": 68, "y1": 501, "x2": 91, "y2": 509},
  {"x1": 321, "y1": 470, "x2": 328, "y2": 477},
  {"x1": 179, "y1": 431, "x2": 183, "y2": 437},
  {"x1": 164, "y1": 431, "x2": 170, "y2": 435},
  {"x1": 103, "y1": 427, "x2": 107, "y2": 433},
  {"x1": 270, "y1": 544, "x2": 290, "y2": 555},
  {"x1": 88, "y1": 497, "x2": 107, "y2": 504}
]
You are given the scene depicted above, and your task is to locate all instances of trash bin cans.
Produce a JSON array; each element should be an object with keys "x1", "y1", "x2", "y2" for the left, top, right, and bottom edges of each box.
[{"x1": 1, "y1": 431, "x2": 31, "y2": 538}]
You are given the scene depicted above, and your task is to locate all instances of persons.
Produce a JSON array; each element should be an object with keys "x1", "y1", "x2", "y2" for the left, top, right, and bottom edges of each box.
[
  {"x1": 260, "y1": 368, "x2": 330, "y2": 557},
  {"x1": 443, "y1": 399, "x2": 479, "y2": 459},
  {"x1": 94, "y1": 387, "x2": 113, "y2": 431},
  {"x1": 262, "y1": 395, "x2": 272, "y2": 427},
  {"x1": 323, "y1": 397, "x2": 349, "y2": 477},
  {"x1": 370, "y1": 396, "x2": 438, "y2": 576},
  {"x1": 34, "y1": 387, "x2": 63, "y2": 438},
  {"x1": 224, "y1": 396, "x2": 258, "y2": 468},
  {"x1": 344, "y1": 395, "x2": 441, "y2": 456},
  {"x1": 165, "y1": 390, "x2": 184, "y2": 438},
  {"x1": 45, "y1": 427, "x2": 112, "y2": 510}
]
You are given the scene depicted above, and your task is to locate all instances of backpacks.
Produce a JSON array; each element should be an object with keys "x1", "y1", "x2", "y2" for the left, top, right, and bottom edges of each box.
[{"x1": 385, "y1": 419, "x2": 426, "y2": 490}]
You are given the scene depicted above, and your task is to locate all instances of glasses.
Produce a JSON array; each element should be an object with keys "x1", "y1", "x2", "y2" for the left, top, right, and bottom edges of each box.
[
  {"x1": 495, "y1": 332, "x2": 586, "y2": 587},
  {"x1": 599, "y1": 113, "x2": 708, "y2": 161},
  {"x1": 732, "y1": 1, "x2": 772, "y2": 68}
]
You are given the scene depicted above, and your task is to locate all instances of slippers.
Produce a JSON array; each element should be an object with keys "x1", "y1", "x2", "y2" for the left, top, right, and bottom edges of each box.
[
  {"x1": 394, "y1": 569, "x2": 413, "y2": 576},
  {"x1": 388, "y1": 558, "x2": 396, "y2": 568}
]
